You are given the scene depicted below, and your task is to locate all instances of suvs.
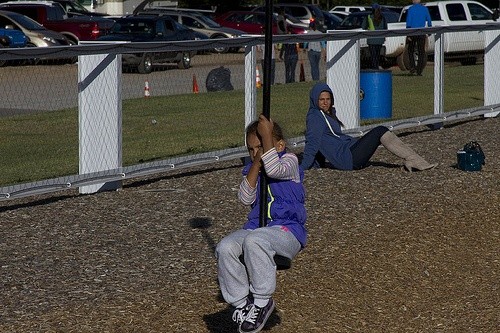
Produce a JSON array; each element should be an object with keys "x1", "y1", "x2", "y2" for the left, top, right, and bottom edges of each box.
[{"x1": 135, "y1": 7, "x2": 248, "y2": 53}]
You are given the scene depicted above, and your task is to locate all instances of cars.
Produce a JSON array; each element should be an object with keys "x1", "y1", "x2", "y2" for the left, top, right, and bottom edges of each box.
[
  {"x1": 0, "y1": 10, "x2": 79, "y2": 66},
  {"x1": 93, "y1": 15, "x2": 201, "y2": 74},
  {"x1": 211, "y1": 0, "x2": 402, "y2": 35}
]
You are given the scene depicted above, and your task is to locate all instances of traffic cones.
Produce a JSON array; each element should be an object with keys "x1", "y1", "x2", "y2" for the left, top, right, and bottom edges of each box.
[
  {"x1": 256, "y1": 66, "x2": 264, "y2": 88},
  {"x1": 144, "y1": 79, "x2": 150, "y2": 98},
  {"x1": 299, "y1": 61, "x2": 305, "y2": 82},
  {"x1": 192, "y1": 74, "x2": 199, "y2": 93}
]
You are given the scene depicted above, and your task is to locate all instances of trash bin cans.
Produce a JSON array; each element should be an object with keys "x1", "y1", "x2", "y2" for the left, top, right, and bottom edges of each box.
[{"x1": 360, "y1": 69, "x2": 392, "y2": 120}]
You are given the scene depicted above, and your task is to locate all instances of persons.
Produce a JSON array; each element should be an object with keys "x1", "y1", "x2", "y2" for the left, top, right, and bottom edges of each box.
[
  {"x1": 300, "y1": 82, "x2": 438, "y2": 172},
  {"x1": 256, "y1": 0, "x2": 432, "y2": 86},
  {"x1": 215, "y1": 113, "x2": 306, "y2": 333}
]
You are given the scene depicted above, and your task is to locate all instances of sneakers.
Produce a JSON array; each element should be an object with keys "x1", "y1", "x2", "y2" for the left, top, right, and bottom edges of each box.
[{"x1": 232, "y1": 298, "x2": 276, "y2": 333}]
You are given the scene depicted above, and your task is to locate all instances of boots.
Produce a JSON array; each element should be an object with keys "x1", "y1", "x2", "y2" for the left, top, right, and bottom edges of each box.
[{"x1": 380, "y1": 130, "x2": 438, "y2": 173}]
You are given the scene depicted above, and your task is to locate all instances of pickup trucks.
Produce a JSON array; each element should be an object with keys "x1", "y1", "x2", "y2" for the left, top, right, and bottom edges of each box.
[
  {"x1": 326, "y1": 0, "x2": 500, "y2": 67},
  {"x1": 0, "y1": 0, "x2": 110, "y2": 45}
]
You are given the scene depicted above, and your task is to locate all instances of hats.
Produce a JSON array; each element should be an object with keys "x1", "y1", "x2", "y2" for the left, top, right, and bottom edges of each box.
[{"x1": 372, "y1": 3, "x2": 380, "y2": 8}]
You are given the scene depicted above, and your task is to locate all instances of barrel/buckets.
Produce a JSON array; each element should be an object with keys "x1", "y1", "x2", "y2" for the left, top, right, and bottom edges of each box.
[{"x1": 360, "y1": 69, "x2": 393, "y2": 119}]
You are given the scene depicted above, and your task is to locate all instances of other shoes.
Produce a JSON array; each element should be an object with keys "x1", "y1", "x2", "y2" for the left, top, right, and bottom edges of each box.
[{"x1": 407, "y1": 69, "x2": 421, "y2": 75}]
